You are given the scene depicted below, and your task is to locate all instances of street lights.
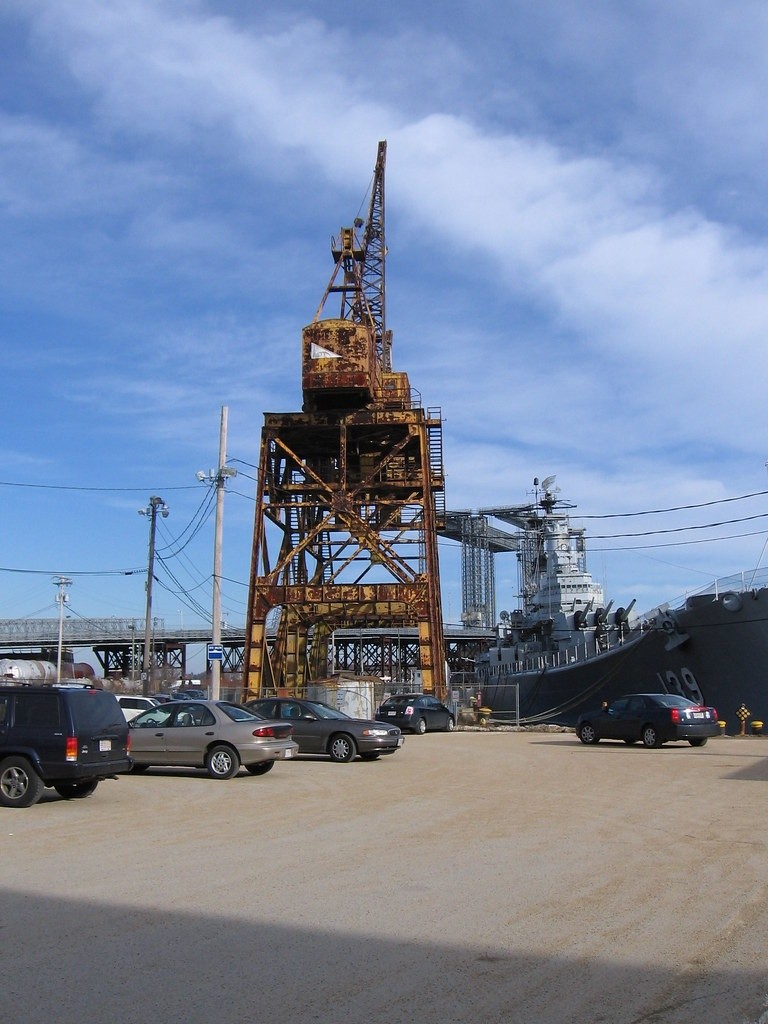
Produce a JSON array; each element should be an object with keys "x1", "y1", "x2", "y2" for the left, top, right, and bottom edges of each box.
[{"x1": 137, "y1": 493, "x2": 169, "y2": 696}]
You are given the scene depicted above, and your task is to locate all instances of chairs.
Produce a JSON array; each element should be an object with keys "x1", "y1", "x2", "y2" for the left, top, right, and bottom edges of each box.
[{"x1": 182, "y1": 713, "x2": 192, "y2": 727}]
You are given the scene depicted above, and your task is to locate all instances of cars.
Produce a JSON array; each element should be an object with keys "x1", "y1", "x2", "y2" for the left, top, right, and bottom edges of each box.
[
  {"x1": 115, "y1": 689, "x2": 205, "y2": 726},
  {"x1": 375, "y1": 692, "x2": 457, "y2": 735},
  {"x1": 126, "y1": 699, "x2": 300, "y2": 781},
  {"x1": 575, "y1": 693, "x2": 723, "y2": 749},
  {"x1": 228, "y1": 697, "x2": 406, "y2": 763}
]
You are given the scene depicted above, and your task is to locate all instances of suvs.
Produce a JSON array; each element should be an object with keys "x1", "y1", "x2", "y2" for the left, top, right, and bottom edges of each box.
[{"x1": 0, "y1": 677, "x2": 134, "y2": 809}]
